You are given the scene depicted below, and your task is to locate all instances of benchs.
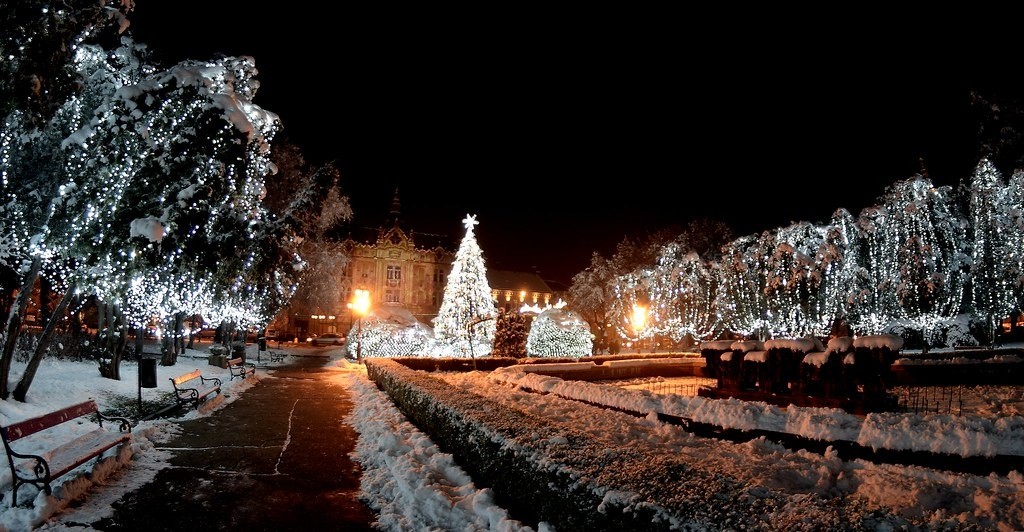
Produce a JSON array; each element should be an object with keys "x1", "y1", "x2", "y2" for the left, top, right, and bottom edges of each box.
[
  {"x1": 0, "y1": 398, "x2": 132, "y2": 509},
  {"x1": 168, "y1": 368, "x2": 222, "y2": 414},
  {"x1": 269, "y1": 350, "x2": 284, "y2": 362},
  {"x1": 226, "y1": 356, "x2": 256, "y2": 382}
]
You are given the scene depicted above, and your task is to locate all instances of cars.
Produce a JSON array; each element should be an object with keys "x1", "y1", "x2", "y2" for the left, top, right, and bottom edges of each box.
[
  {"x1": 196, "y1": 327, "x2": 237, "y2": 338},
  {"x1": 313, "y1": 333, "x2": 346, "y2": 346},
  {"x1": 654, "y1": 339, "x2": 670, "y2": 347}
]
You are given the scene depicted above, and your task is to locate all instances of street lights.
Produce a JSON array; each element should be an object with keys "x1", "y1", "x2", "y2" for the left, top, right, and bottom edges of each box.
[{"x1": 348, "y1": 287, "x2": 371, "y2": 364}]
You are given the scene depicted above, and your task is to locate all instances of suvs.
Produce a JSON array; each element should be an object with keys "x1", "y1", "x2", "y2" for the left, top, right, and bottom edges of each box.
[{"x1": 265, "y1": 329, "x2": 278, "y2": 340}]
